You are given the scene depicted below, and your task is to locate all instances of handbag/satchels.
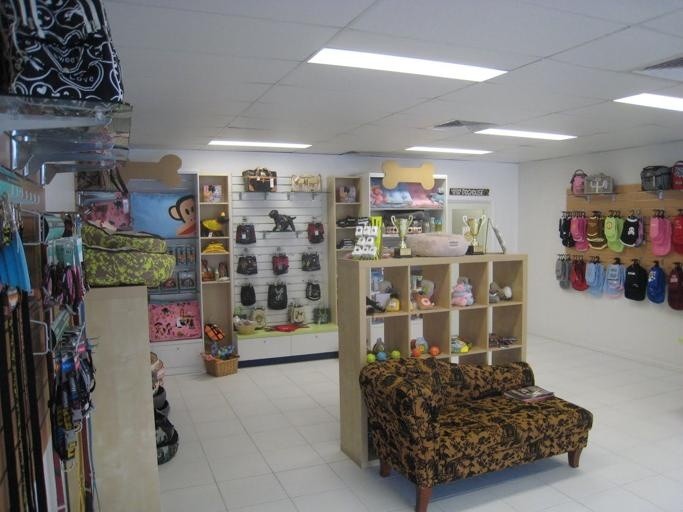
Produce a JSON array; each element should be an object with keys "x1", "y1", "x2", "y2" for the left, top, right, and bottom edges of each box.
[
  {"x1": 291, "y1": 173, "x2": 322, "y2": 193},
  {"x1": 1, "y1": 1, "x2": 125, "y2": 113},
  {"x1": 243, "y1": 168, "x2": 278, "y2": 193},
  {"x1": 584, "y1": 172, "x2": 616, "y2": 195}
]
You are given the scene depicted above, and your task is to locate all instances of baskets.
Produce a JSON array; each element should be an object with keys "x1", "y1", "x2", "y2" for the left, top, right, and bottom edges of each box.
[{"x1": 199, "y1": 350, "x2": 242, "y2": 377}]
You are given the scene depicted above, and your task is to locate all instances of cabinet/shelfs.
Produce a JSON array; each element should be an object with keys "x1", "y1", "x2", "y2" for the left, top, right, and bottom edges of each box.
[
  {"x1": 63, "y1": 281, "x2": 163, "y2": 511},
  {"x1": 238, "y1": 320, "x2": 339, "y2": 368},
  {"x1": 362, "y1": 173, "x2": 452, "y2": 252},
  {"x1": 334, "y1": 250, "x2": 532, "y2": 467},
  {"x1": 326, "y1": 171, "x2": 361, "y2": 324},
  {"x1": 192, "y1": 172, "x2": 236, "y2": 362}
]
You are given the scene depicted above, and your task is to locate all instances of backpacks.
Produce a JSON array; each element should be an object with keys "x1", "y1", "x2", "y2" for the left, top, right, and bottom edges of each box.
[
  {"x1": 80, "y1": 216, "x2": 177, "y2": 290},
  {"x1": 570, "y1": 168, "x2": 587, "y2": 196},
  {"x1": 672, "y1": 160, "x2": 683, "y2": 190},
  {"x1": 640, "y1": 164, "x2": 671, "y2": 191}
]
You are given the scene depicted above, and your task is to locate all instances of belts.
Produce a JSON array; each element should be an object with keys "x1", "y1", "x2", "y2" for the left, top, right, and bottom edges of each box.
[{"x1": 0, "y1": 191, "x2": 50, "y2": 512}]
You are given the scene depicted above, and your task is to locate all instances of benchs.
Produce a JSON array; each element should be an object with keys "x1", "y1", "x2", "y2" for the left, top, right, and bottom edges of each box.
[{"x1": 357, "y1": 359, "x2": 593, "y2": 511}]
